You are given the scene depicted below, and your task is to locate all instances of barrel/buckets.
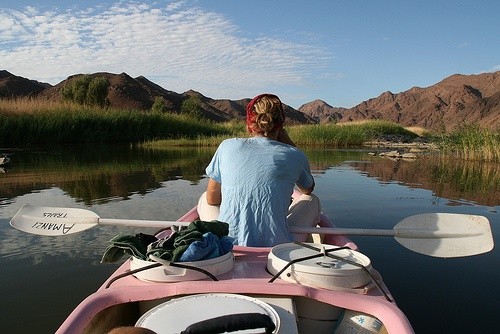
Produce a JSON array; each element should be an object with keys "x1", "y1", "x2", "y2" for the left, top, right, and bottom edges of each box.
[
  {"x1": 129, "y1": 252, "x2": 235, "y2": 319},
  {"x1": 266, "y1": 242, "x2": 372, "y2": 334},
  {"x1": 333, "y1": 309, "x2": 383, "y2": 334}
]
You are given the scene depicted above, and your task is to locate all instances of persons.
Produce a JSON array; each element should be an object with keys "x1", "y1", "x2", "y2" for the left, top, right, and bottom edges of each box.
[{"x1": 204, "y1": 93, "x2": 325, "y2": 247}]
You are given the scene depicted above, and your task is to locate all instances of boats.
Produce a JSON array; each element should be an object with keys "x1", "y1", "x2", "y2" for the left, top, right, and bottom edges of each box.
[{"x1": 54, "y1": 204, "x2": 419, "y2": 333}]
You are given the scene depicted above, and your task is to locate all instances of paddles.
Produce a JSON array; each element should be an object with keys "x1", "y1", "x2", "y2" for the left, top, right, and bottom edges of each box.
[{"x1": 10, "y1": 203, "x2": 495, "y2": 259}]
